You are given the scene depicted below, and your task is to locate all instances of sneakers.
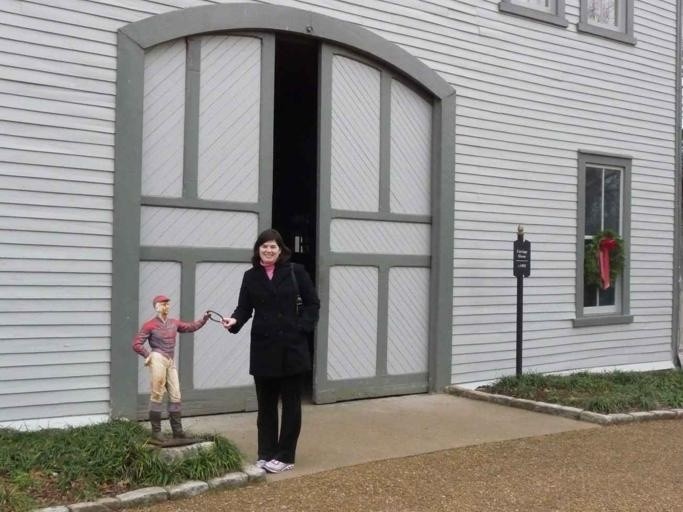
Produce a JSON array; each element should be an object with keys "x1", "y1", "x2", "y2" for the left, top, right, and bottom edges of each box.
[
  {"x1": 264, "y1": 459, "x2": 295, "y2": 474},
  {"x1": 255, "y1": 459, "x2": 268, "y2": 469}
]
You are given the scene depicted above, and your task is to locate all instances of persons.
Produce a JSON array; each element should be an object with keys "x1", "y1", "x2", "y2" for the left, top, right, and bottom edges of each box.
[
  {"x1": 220, "y1": 229, "x2": 320, "y2": 473},
  {"x1": 133, "y1": 295, "x2": 211, "y2": 442}
]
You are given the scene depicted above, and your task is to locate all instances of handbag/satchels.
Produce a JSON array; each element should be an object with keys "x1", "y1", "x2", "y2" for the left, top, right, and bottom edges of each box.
[{"x1": 289, "y1": 261, "x2": 304, "y2": 315}]
